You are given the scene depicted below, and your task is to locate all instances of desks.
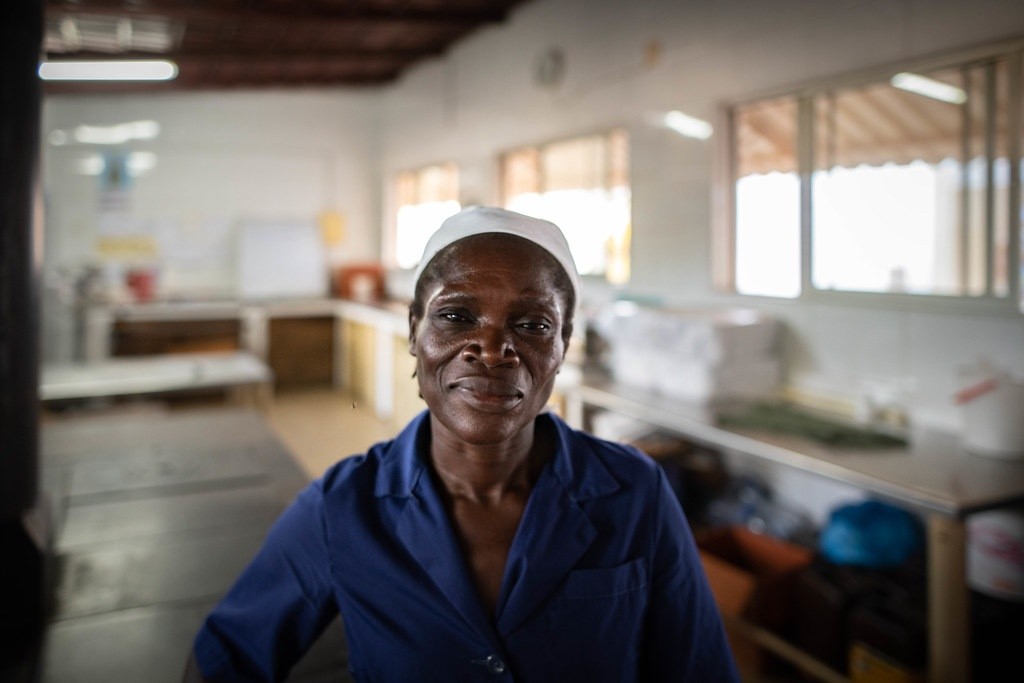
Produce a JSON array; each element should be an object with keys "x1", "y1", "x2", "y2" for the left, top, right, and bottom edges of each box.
[
  {"x1": 90, "y1": 302, "x2": 254, "y2": 403},
  {"x1": 249, "y1": 297, "x2": 337, "y2": 359},
  {"x1": 562, "y1": 385, "x2": 1024, "y2": 683}
]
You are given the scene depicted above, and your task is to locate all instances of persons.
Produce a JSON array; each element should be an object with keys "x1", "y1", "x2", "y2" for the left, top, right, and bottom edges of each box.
[{"x1": 176, "y1": 207, "x2": 743, "y2": 683}]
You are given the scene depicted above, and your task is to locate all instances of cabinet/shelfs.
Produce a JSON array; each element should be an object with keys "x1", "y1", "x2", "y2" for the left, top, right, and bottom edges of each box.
[{"x1": 339, "y1": 298, "x2": 590, "y2": 426}]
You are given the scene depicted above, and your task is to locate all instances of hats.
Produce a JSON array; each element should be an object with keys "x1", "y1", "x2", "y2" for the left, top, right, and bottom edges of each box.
[{"x1": 410, "y1": 206, "x2": 580, "y2": 334}]
